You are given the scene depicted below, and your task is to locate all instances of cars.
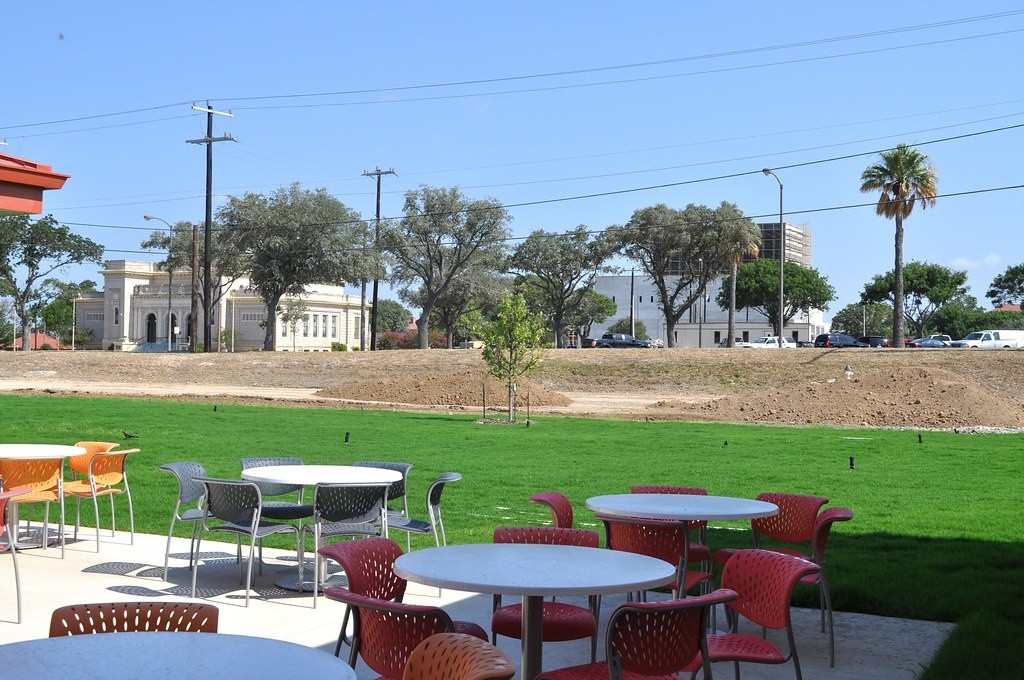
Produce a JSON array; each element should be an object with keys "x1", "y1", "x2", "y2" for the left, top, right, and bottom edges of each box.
[
  {"x1": 798, "y1": 340, "x2": 815, "y2": 348},
  {"x1": 909, "y1": 337, "x2": 951, "y2": 348},
  {"x1": 927, "y1": 334, "x2": 952, "y2": 346},
  {"x1": 814, "y1": 333, "x2": 870, "y2": 348},
  {"x1": 857, "y1": 336, "x2": 892, "y2": 348}
]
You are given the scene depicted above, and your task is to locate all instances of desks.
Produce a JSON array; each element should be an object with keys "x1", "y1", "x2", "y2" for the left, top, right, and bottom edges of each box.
[
  {"x1": 242, "y1": 464, "x2": 413, "y2": 593},
  {"x1": 4, "y1": 444, "x2": 91, "y2": 552},
  {"x1": 0, "y1": 631, "x2": 355, "y2": 680},
  {"x1": 393, "y1": 542, "x2": 678, "y2": 680},
  {"x1": 584, "y1": 493, "x2": 779, "y2": 649}
]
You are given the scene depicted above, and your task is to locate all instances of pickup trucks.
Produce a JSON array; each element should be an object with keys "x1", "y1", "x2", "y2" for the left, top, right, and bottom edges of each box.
[
  {"x1": 582, "y1": 333, "x2": 654, "y2": 348},
  {"x1": 741, "y1": 336, "x2": 796, "y2": 349},
  {"x1": 951, "y1": 331, "x2": 1018, "y2": 349}
]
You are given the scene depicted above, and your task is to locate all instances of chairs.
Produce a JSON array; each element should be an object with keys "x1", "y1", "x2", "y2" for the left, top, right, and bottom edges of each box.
[
  {"x1": 0, "y1": 440, "x2": 142, "y2": 628},
  {"x1": 49, "y1": 601, "x2": 221, "y2": 634},
  {"x1": 317, "y1": 526, "x2": 823, "y2": 680},
  {"x1": 161, "y1": 456, "x2": 463, "y2": 613},
  {"x1": 531, "y1": 484, "x2": 853, "y2": 669}
]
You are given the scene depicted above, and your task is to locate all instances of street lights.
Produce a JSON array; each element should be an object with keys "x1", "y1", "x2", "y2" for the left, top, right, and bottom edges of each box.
[
  {"x1": 763, "y1": 168, "x2": 784, "y2": 349},
  {"x1": 144, "y1": 215, "x2": 173, "y2": 351},
  {"x1": 72, "y1": 293, "x2": 81, "y2": 350}
]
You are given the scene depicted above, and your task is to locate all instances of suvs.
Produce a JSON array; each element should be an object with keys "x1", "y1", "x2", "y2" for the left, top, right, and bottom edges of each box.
[{"x1": 718, "y1": 337, "x2": 744, "y2": 348}]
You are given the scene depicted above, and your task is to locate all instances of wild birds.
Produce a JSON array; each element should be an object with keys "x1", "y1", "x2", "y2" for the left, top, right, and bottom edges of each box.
[
  {"x1": 724, "y1": 440, "x2": 728, "y2": 445},
  {"x1": 122, "y1": 431, "x2": 136, "y2": 439}
]
